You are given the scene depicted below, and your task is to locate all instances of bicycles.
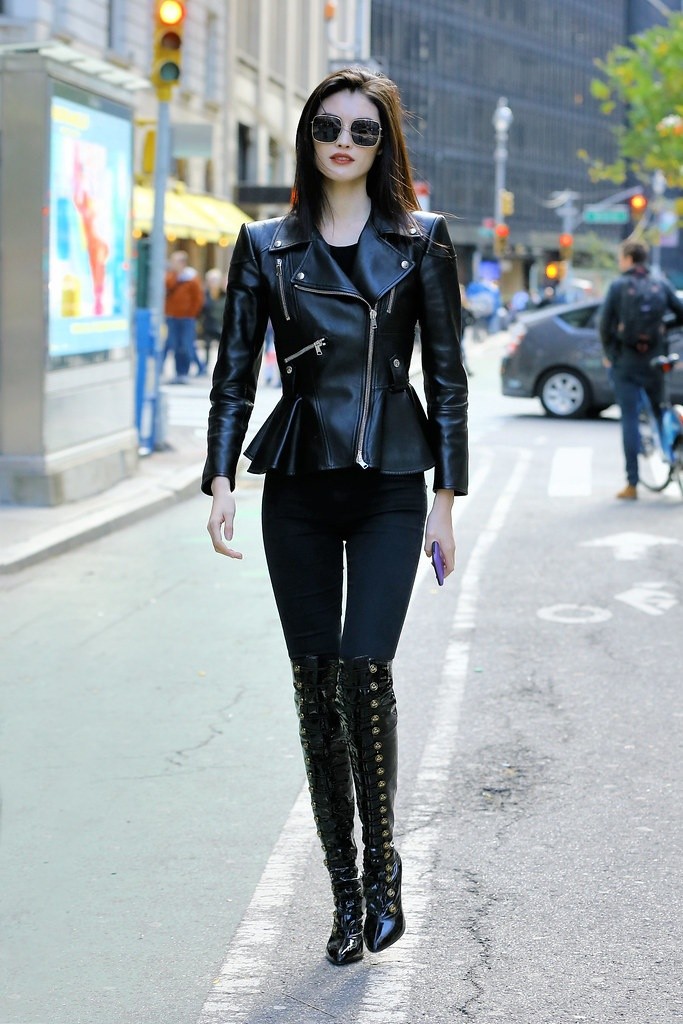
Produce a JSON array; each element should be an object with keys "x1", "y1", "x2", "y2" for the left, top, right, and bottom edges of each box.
[{"x1": 605, "y1": 322, "x2": 683, "y2": 496}]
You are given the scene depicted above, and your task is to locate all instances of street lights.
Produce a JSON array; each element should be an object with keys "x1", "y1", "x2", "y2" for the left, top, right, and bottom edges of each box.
[{"x1": 489, "y1": 95, "x2": 512, "y2": 261}]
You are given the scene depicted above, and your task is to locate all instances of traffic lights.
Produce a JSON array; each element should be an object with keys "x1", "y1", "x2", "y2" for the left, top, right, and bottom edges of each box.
[
  {"x1": 150, "y1": 0, "x2": 188, "y2": 91},
  {"x1": 496, "y1": 188, "x2": 516, "y2": 218},
  {"x1": 631, "y1": 195, "x2": 647, "y2": 220},
  {"x1": 557, "y1": 232, "x2": 574, "y2": 259},
  {"x1": 546, "y1": 261, "x2": 563, "y2": 281}
]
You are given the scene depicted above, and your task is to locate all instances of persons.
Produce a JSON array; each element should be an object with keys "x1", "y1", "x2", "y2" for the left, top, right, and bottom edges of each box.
[
  {"x1": 197, "y1": 69, "x2": 467, "y2": 967},
  {"x1": 163, "y1": 250, "x2": 210, "y2": 384},
  {"x1": 458, "y1": 282, "x2": 476, "y2": 377},
  {"x1": 600, "y1": 238, "x2": 683, "y2": 502},
  {"x1": 201, "y1": 267, "x2": 226, "y2": 367}
]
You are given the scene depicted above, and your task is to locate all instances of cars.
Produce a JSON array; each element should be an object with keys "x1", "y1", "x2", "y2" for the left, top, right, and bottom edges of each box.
[{"x1": 497, "y1": 286, "x2": 683, "y2": 421}]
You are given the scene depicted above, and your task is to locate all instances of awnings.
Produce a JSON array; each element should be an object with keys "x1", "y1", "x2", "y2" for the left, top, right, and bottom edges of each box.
[{"x1": 130, "y1": 185, "x2": 257, "y2": 248}]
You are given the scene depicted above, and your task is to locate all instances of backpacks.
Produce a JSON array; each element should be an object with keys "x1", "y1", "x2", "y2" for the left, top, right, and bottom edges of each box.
[{"x1": 610, "y1": 274, "x2": 664, "y2": 357}]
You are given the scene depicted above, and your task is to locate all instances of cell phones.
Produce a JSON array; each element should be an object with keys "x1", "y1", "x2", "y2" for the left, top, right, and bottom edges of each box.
[{"x1": 431, "y1": 541, "x2": 444, "y2": 586}]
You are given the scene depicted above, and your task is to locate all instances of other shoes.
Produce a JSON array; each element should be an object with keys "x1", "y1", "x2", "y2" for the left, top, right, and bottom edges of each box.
[{"x1": 618, "y1": 484, "x2": 638, "y2": 501}]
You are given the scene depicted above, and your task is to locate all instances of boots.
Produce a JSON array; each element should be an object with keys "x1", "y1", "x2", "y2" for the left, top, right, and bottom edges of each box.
[
  {"x1": 336, "y1": 656, "x2": 404, "y2": 953},
  {"x1": 289, "y1": 656, "x2": 365, "y2": 964}
]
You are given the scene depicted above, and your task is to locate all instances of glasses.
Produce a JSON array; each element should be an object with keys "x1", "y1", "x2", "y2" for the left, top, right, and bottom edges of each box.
[{"x1": 308, "y1": 115, "x2": 383, "y2": 148}]
